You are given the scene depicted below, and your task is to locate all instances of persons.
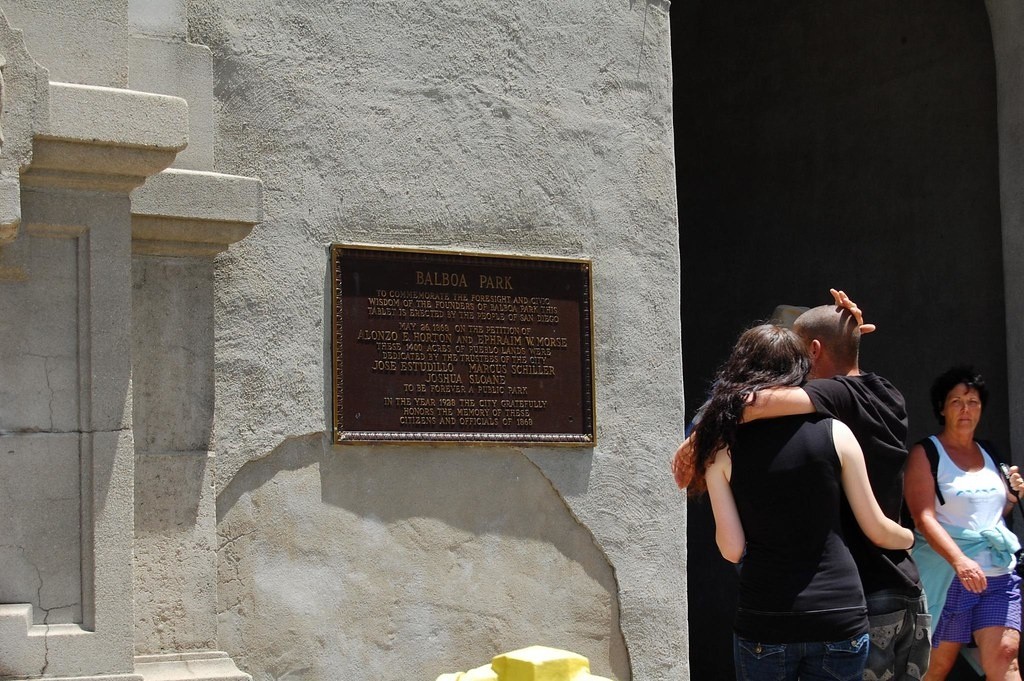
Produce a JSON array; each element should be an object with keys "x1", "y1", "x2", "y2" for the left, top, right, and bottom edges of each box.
[
  {"x1": 897, "y1": 366, "x2": 1024, "y2": 681},
  {"x1": 690, "y1": 323, "x2": 917, "y2": 680},
  {"x1": 671, "y1": 288, "x2": 931, "y2": 681}
]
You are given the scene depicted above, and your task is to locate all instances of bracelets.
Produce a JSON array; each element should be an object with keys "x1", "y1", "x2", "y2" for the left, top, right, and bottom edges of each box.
[{"x1": 905, "y1": 527, "x2": 915, "y2": 550}]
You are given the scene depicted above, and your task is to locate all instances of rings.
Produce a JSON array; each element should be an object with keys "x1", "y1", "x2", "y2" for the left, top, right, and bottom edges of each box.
[{"x1": 966, "y1": 577, "x2": 971, "y2": 580}]
